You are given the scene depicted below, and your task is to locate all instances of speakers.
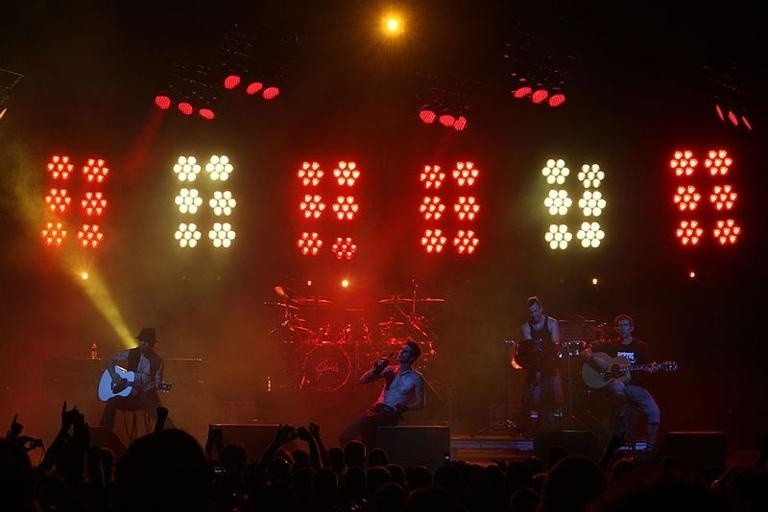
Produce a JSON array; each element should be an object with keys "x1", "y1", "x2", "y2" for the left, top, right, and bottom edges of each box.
[
  {"x1": 652, "y1": 432, "x2": 728, "y2": 477},
  {"x1": 52, "y1": 425, "x2": 125, "y2": 467},
  {"x1": 368, "y1": 425, "x2": 450, "y2": 469},
  {"x1": 675, "y1": 417, "x2": 706, "y2": 432},
  {"x1": 573, "y1": 416, "x2": 609, "y2": 448},
  {"x1": 207, "y1": 423, "x2": 294, "y2": 464},
  {"x1": 534, "y1": 430, "x2": 599, "y2": 459}
]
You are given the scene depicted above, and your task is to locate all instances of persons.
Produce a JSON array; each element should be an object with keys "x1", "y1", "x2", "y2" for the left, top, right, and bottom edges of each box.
[
  {"x1": 100, "y1": 327, "x2": 169, "y2": 427},
  {"x1": 580, "y1": 313, "x2": 660, "y2": 452},
  {"x1": 1, "y1": 400, "x2": 768, "y2": 512},
  {"x1": 522, "y1": 297, "x2": 564, "y2": 402},
  {"x1": 340, "y1": 341, "x2": 425, "y2": 447}
]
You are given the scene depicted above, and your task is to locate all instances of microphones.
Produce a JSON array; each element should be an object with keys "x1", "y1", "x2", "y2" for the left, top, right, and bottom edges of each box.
[{"x1": 373, "y1": 349, "x2": 394, "y2": 376}]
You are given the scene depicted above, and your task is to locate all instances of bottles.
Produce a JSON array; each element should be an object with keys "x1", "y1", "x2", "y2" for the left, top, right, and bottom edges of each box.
[{"x1": 91, "y1": 341, "x2": 97, "y2": 361}]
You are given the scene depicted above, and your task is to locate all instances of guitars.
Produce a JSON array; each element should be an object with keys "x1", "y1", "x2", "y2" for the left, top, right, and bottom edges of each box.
[
  {"x1": 582, "y1": 353, "x2": 679, "y2": 389},
  {"x1": 98, "y1": 366, "x2": 171, "y2": 402}
]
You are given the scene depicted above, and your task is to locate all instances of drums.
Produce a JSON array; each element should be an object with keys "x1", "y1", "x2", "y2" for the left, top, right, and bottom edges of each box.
[{"x1": 303, "y1": 339, "x2": 352, "y2": 393}]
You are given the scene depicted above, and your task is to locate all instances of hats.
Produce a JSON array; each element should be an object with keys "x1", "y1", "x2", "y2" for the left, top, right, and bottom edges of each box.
[{"x1": 135, "y1": 328, "x2": 156, "y2": 341}]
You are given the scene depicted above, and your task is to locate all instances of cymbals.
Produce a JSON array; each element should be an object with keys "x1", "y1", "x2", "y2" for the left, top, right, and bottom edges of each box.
[
  {"x1": 420, "y1": 299, "x2": 443, "y2": 303},
  {"x1": 263, "y1": 301, "x2": 298, "y2": 313},
  {"x1": 302, "y1": 298, "x2": 331, "y2": 306},
  {"x1": 381, "y1": 298, "x2": 410, "y2": 304},
  {"x1": 378, "y1": 322, "x2": 405, "y2": 326}
]
